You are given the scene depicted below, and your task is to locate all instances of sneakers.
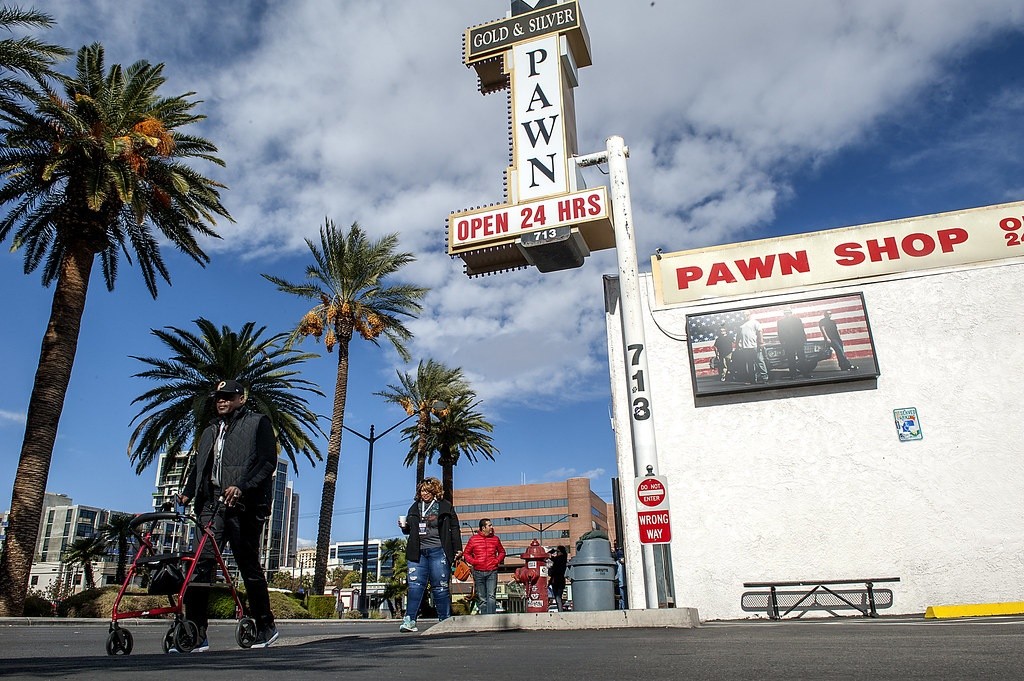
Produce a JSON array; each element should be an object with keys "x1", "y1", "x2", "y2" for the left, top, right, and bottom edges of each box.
[
  {"x1": 169, "y1": 626, "x2": 209, "y2": 655},
  {"x1": 399, "y1": 615, "x2": 418, "y2": 632},
  {"x1": 250, "y1": 612, "x2": 279, "y2": 648}
]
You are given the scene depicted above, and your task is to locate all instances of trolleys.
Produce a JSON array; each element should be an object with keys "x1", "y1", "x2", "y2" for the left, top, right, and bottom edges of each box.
[{"x1": 105, "y1": 492, "x2": 259, "y2": 659}]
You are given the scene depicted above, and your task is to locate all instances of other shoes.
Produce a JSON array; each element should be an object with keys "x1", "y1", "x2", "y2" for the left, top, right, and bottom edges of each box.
[{"x1": 850, "y1": 365, "x2": 858, "y2": 370}]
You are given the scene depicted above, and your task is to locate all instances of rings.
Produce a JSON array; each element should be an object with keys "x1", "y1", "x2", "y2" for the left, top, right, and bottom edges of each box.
[{"x1": 234, "y1": 495, "x2": 239, "y2": 498}]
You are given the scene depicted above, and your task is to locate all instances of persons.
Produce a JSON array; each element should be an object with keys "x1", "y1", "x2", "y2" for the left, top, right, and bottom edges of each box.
[
  {"x1": 397, "y1": 476, "x2": 464, "y2": 632},
  {"x1": 547, "y1": 545, "x2": 568, "y2": 612},
  {"x1": 336, "y1": 599, "x2": 344, "y2": 619},
  {"x1": 712, "y1": 326, "x2": 734, "y2": 382},
  {"x1": 463, "y1": 519, "x2": 506, "y2": 614},
  {"x1": 615, "y1": 549, "x2": 628, "y2": 609},
  {"x1": 819, "y1": 309, "x2": 859, "y2": 371},
  {"x1": 777, "y1": 310, "x2": 815, "y2": 381},
  {"x1": 168, "y1": 379, "x2": 279, "y2": 654},
  {"x1": 734, "y1": 309, "x2": 769, "y2": 385}
]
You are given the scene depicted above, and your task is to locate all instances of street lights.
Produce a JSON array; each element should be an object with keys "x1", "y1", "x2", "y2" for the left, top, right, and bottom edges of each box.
[
  {"x1": 304, "y1": 401, "x2": 447, "y2": 619},
  {"x1": 504, "y1": 513, "x2": 579, "y2": 546},
  {"x1": 287, "y1": 554, "x2": 315, "y2": 579}
]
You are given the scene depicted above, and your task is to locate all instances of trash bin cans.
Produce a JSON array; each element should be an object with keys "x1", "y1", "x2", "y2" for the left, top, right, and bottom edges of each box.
[{"x1": 564, "y1": 537, "x2": 619, "y2": 611}]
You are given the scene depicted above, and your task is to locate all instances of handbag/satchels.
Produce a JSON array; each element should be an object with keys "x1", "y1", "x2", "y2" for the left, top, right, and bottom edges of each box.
[
  {"x1": 454, "y1": 558, "x2": 475, "y2": 600},
  {"x1": 548, "y1": 568, "x2": 555, "y2": 576},
  {"x1": 149, "y1": 561, "x2": 184, "y2": 594}
]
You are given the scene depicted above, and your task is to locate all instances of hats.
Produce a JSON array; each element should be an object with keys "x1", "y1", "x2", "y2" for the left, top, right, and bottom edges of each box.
[
  {"x1": 617, "y1": 551, "x2": 624, "y2": 559},
  {"x1": 211, "y1": 380, "x2": 244, "y2": 395}
]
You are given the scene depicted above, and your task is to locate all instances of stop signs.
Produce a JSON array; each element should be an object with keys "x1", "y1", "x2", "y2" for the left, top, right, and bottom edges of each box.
[{"x1": 634, "y1": 475, "x2": 670, "y2": 513}]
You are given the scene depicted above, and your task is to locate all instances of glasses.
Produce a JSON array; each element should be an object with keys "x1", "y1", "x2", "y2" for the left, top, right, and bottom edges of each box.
[
  {"x1": 421, "y1": 480, "x2": 434, "y2": 485},
  {"x1": 557, "y1": 551, "x2": 561, "y2": 553}
]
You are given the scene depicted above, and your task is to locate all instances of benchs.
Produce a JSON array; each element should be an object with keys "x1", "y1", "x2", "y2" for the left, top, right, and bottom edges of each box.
[{"x1": 741, "y1": 577, "x2": 901, "y2": 622}]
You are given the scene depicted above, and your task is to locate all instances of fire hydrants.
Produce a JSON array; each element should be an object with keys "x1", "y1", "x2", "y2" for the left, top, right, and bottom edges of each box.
[{"x1": 513, "y1": 537, "x2": 551, "y2": 612}]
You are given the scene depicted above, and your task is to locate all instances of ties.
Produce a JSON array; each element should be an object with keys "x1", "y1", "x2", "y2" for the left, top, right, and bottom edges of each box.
[{"x1": 213, "y1": 421, "x2": 226, "y2": 478}]
